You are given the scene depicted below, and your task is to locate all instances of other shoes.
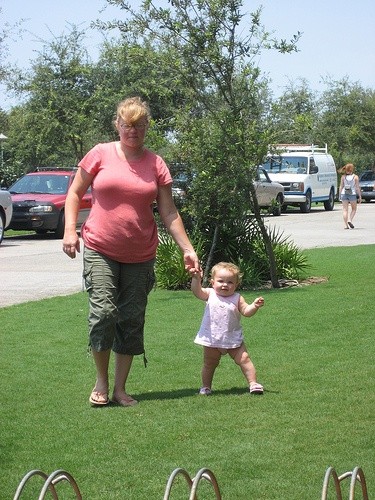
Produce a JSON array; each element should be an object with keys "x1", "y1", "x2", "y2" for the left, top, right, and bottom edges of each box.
[
  {"x1": 348, "y1": 222, "x2": 354, "y2": 228},
  {"x1": 200, "y1": 387, "x2": 212, "y2": 395},
  {"x1": 344, "y1": 227, "x2": 349, "y2": 229},
  {"x1": 249, "y1": 383, "x2": 263, "y2": 394}
]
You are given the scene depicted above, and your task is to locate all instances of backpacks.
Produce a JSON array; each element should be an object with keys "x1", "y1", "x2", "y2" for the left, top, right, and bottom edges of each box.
[{"x1": 345, "y1": 176, "x2": 355, "y2": 195}]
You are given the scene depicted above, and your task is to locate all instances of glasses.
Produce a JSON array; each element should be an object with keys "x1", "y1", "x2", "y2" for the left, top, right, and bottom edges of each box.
[{"x1": 120, "y1": 123, "x2": 146, "y2": 131}]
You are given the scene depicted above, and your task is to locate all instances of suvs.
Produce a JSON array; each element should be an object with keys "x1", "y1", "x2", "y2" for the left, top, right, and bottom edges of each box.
[
  {"x1": 250, "y1": 165, "x2": 284, "y2": 216},
  {"x1": 4, "y1": 167, "x2": 92, "y2": 239},
  {"x1": 356, "y1": 170, "x2": 375, "y2": 203}
]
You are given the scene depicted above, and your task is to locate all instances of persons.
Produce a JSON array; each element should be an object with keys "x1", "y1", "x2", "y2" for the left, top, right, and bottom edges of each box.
[
  {"x1": 191, "y1": 262, "x2": 264, "y2": 395},
  {"x1": 63, "y1": 97, "x2": 203, "y2": 406},
  {"x1": 338, "y1": 164, "x2": 362, "y2": 229}
]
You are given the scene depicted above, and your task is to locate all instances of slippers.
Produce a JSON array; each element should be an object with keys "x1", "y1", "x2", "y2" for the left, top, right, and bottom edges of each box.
[
  {"x1": 89, "y1": 390, "x2": 109, "y2": 404},
  {"x1": 112, "y1": 394, "x2": 137, "y2": 406}
]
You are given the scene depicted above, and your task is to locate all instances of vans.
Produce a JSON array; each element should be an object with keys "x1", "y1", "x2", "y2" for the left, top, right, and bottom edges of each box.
[{"x1": 259, "y1": 144, "x2": 337, "y2": 213}]
